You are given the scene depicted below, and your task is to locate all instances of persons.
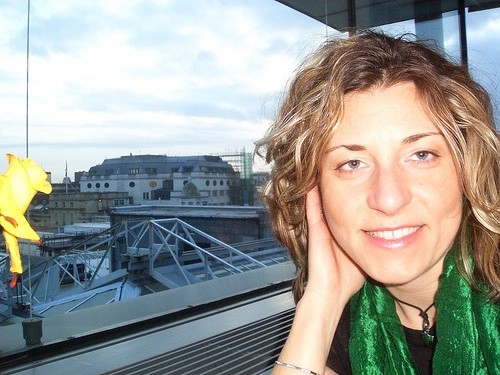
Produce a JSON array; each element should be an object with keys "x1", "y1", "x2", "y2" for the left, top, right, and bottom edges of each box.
[{"x1": 252, "y1": 21, "x2": 500, "y2": 375}]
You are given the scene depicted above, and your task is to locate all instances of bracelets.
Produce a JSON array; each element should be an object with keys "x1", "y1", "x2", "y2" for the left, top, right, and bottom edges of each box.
[{"x1": 274, "y1": 360, "x2": 321, "y2": 375}]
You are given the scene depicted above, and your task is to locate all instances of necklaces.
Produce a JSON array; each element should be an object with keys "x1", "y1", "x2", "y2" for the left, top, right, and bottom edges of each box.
[{"x1": 391, "y1": 292, "x2": 435, "y2": 344}]
